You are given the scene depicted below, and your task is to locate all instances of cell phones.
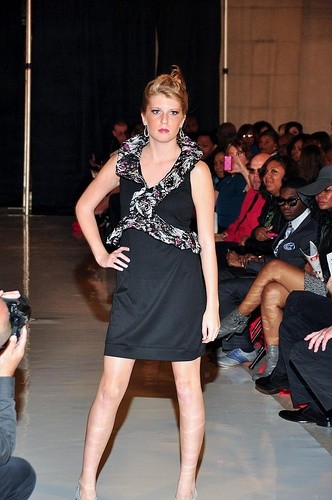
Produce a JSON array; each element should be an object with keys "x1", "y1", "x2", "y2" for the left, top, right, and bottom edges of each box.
[
  {"x1": 224, "y1": 156, "x2": 232, "y2": 170},
  {"x1": 266, "y1": 232, "x2": 278, "y2": 237}
]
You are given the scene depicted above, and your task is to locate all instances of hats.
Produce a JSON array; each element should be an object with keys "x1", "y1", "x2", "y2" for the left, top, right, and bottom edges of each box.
[{"x1": 297, "y1": 165, "x2": 332, "y2": 207}]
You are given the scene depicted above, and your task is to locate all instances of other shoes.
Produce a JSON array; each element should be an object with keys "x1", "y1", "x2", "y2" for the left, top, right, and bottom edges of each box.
[
  {"x1": 175, "y1": 488, "x2": 197, "y2": 500},
  {"x1": 217, "y1": 348, "x2": 258, "y2": 367},
  {"x1": 75, "y1": 485, "x2": 98, "y2": 500},
  {"x1": 278, "y1": 403, "x2": 328, "y2": 427},
  {"x1": 254, "y1": 367, "x2": 290, "y2": 395}
]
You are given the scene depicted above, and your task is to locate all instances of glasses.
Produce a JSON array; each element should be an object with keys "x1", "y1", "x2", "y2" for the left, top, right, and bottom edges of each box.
[
  {"x1": 275, "y1": 197, "x2": 300, "y2": 207},
  {"x1": 242, "y1": 133, "x2": 254, "y2": 138},
  {"x1": 113, "y1": 129, "x2": 129, "y2": 136},
  {"x1": 248, "y1": 168, "x2": 261, "y2": 175}
]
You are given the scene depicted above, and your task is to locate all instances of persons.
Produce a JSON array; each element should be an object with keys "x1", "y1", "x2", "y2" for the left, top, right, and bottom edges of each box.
[
  {"x1": 75, "y1": 68, "x2": 222, "y2": 500},
  {"x1": 0, "y1": 290, "x2": 37, "y2": 500},
  {"x1": 82, "y1": 118, "x2": 332, "y2": 427}
]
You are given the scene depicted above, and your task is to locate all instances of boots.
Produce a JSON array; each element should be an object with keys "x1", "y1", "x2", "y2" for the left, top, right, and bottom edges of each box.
[
  {"x1": 252, "y1": 345, "x2": 279, "y2": 381},
  {"x1": 217, "y1": 307, "x2": 251, "y2": 340}
]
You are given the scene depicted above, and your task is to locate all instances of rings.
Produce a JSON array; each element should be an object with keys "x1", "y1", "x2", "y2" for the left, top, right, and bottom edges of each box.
[{"x1": 318, "y1": 335, "x2": 324, "y2": 341}]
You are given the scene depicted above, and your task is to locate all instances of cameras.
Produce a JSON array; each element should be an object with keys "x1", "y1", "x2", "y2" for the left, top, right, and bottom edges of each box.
[{"x1": 3, "y1": 291, "x2": 31, "y2": 337}]
